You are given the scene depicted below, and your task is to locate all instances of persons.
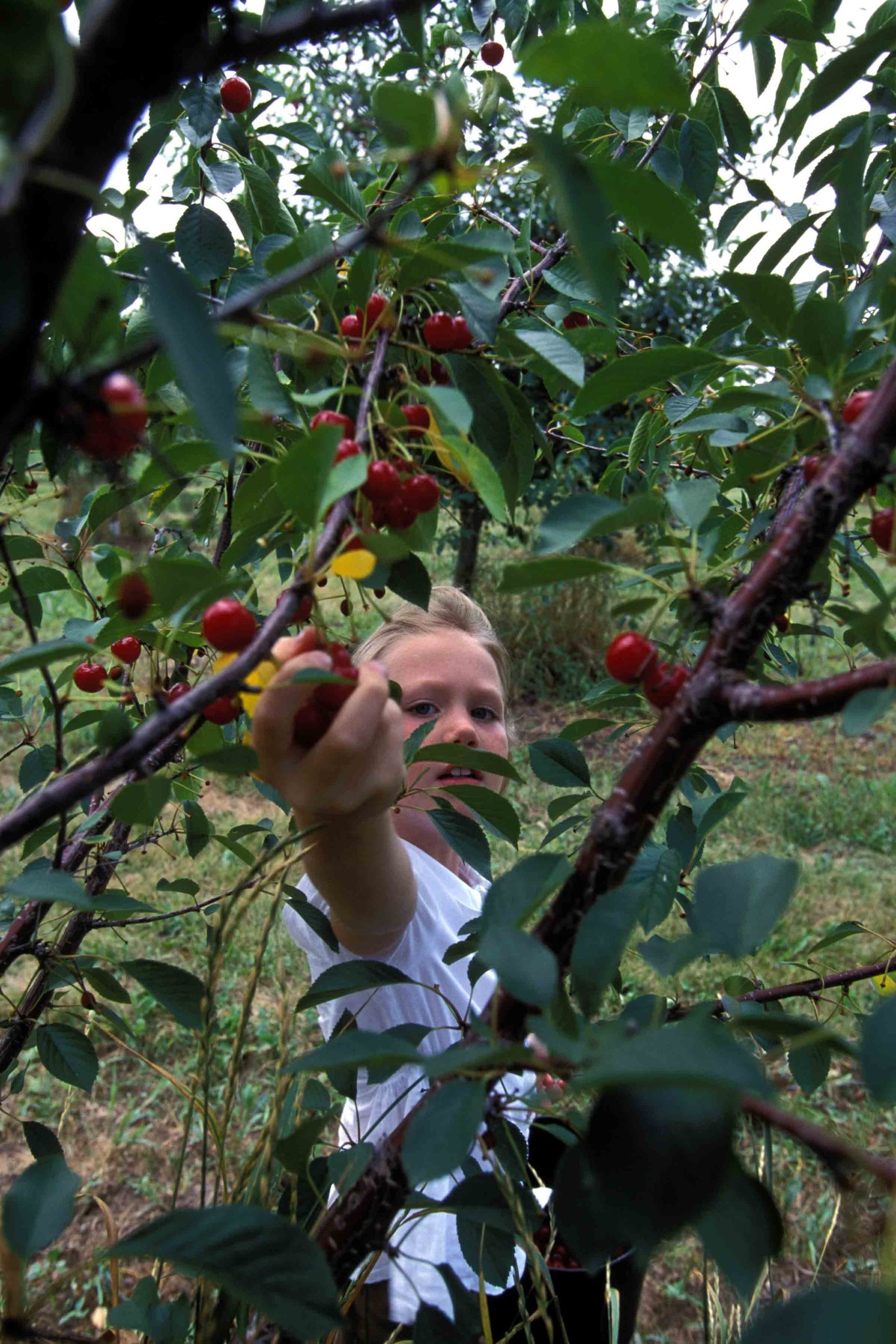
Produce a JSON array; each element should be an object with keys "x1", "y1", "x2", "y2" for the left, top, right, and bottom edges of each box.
[{"x1": 251, "y1": 584, "x2": 587, "y2": 1344}]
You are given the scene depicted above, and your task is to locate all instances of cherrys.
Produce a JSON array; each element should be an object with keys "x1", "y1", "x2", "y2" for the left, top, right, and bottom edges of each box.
[{"x1": 77, "y1": 38, "x2": 895, "y2": 749}]
